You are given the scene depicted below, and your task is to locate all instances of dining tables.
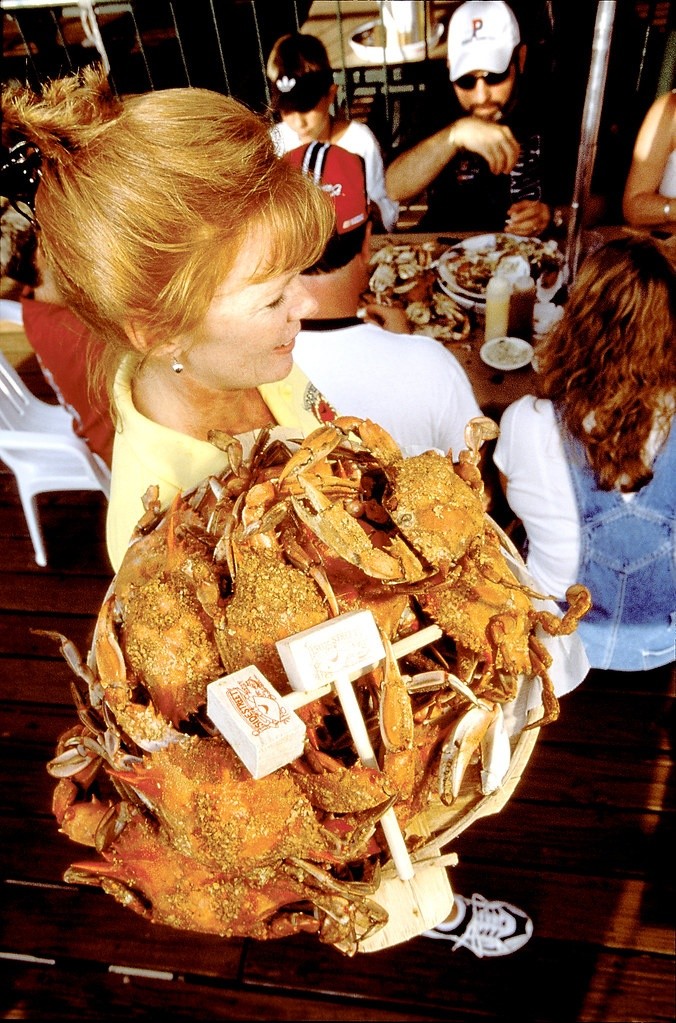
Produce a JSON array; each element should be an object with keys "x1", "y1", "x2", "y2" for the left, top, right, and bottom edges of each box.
[
  {"x1": 365, "y1": 231, "x2": 543, "y2": 406},
  {"x1": 1, "y1": 275, "x2": 35, "y2": 371}
]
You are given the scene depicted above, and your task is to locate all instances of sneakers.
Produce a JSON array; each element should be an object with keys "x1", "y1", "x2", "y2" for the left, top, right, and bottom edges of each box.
[{"x1": 419, "y1": 894, "x2": 532, "y2": 958}]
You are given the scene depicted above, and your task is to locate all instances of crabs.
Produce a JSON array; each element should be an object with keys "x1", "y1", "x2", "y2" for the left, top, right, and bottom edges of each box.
[
  {"x1": 30, "y1": 416, "x2": 592, "y2": 957},
  {"x1": 368, "y1": 239, "x2": 472, "y2": 343}
]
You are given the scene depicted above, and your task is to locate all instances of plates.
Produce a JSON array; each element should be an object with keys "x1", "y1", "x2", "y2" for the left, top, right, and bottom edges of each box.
[{"x1": 479, "y1": 336, "x2": 535, "y2": 371}]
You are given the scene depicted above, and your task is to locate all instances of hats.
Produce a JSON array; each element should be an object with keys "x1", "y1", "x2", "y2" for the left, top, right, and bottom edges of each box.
[
  {"x1": 447, "y1": 1, "x2": 522, "y2": 83},
  {"x1": 277, "y1": 141, "x2": 372, "y2": 240},
  {"x1": 267, "y1": 70, "x2": 331, "y2": 113}
]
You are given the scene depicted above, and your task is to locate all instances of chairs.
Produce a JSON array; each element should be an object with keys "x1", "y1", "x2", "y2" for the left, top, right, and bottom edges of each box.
[{"x1": 0, "y1": 349, "x2": 113, "y2": 566}]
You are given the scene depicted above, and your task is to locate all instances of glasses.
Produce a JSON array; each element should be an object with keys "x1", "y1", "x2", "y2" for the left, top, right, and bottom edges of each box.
[{"x1": 459, "y1": 55, "x2": 516, "y2": 89}]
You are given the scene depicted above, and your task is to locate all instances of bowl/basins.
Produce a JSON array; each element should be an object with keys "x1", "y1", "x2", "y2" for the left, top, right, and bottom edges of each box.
[{"x1": 438, "y1": 233, "x2": 569, "y2": 316}]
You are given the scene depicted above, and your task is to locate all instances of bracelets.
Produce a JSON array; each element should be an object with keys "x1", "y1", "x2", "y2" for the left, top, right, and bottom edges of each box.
[
  {"x1": 553, "y1": 209, "x2": 563, "y2": 226},
  {"x1": 664, "y1": 199, "x2": 671, "y2": 220}
]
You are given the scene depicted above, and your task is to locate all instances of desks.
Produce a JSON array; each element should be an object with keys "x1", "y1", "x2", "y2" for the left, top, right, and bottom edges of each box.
[{"x1": 299, "y1": 1, "x2": 425, "y2": 64}]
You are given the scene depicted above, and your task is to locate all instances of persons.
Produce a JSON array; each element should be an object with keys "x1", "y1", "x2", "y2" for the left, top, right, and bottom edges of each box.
[
  {"x1": 383, "y1": 1, "x2": 605, "y2": 237},
  {"x1": 1, "y1": 64, "x2": 534, "y2": 957},
  {"x1": 494, "y1": 238, "x2": 676, "y2": 674},
  {"x1": 0, "y1": 195, "x2": 116, "y2": 466},
  {"x1": 624, "y1": 87, "x2": 676, "y2": 228},
  {"x1": 285, "y1": 142, "x2": 487, "y2": 472},
  {"x1": 270, "y1": 32, "x2": 399, "y2": 233}
]
